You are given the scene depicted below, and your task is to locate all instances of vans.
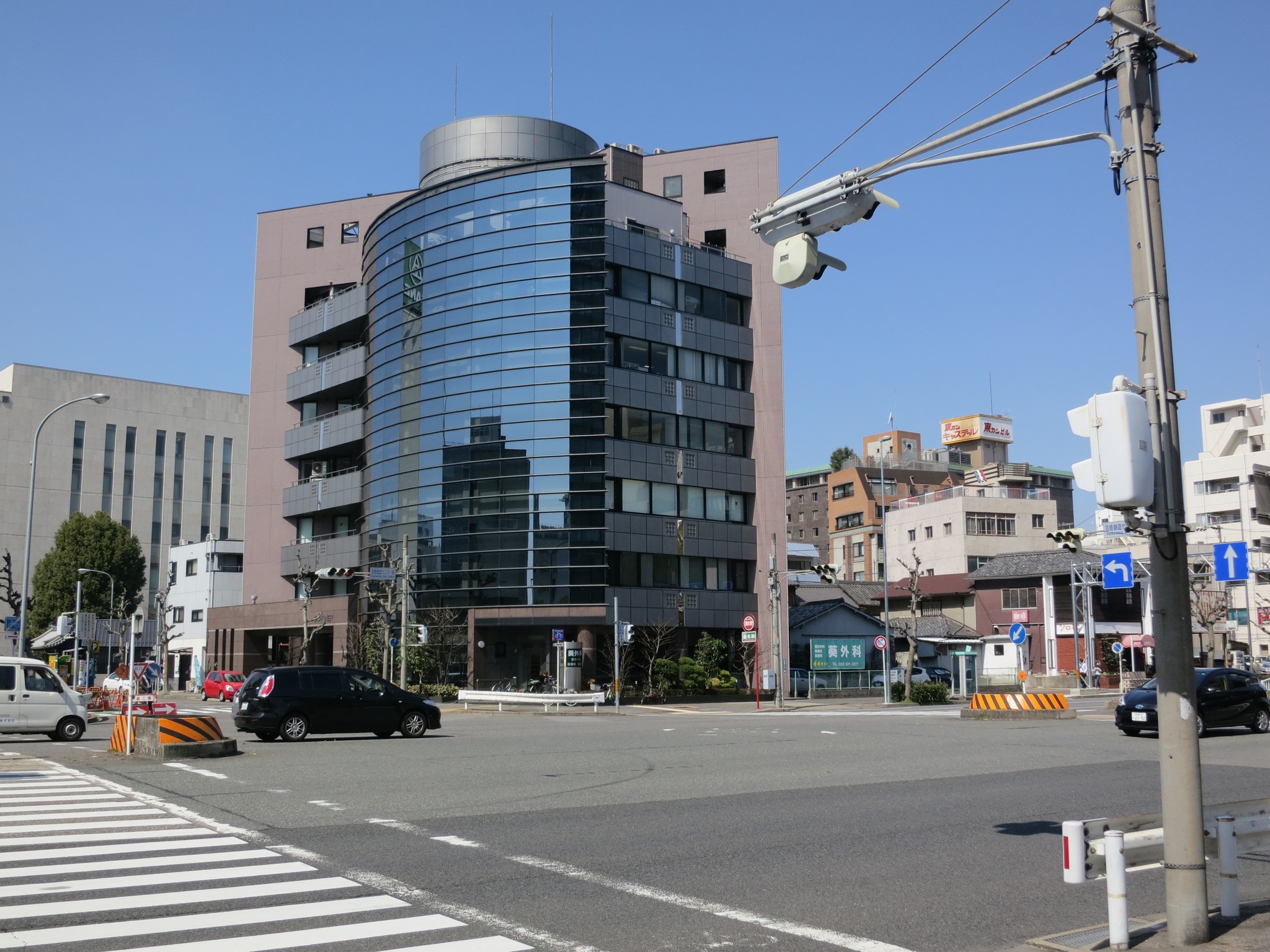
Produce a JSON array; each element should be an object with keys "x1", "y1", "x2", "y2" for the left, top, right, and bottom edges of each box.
[{"x1": 0, "y1": 656, "x2": 91, "y2": 744}]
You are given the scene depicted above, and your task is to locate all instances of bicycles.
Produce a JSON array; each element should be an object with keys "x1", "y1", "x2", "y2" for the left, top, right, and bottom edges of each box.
[
  {"x1": 523, "y1": 676, "x2": 578, "y2": 706},
  {"x1": 640, "y1": 682, "x2": 667, "y2": 704},
  {"x1": 491, "y1": 676, "x2": 518, "y2": 692},
  {"x1": 592, "y1": 682, "x2": 617, "y2": 706}
]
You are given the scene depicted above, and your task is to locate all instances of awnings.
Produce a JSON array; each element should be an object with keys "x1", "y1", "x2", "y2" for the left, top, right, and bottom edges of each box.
[{"x1": 1122, "y1": 634, "x2": 1155, "y2": 649}]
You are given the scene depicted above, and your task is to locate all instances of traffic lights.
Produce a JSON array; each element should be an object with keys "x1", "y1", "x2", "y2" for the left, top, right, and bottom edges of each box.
[
  {"x1": 1047, "y1": 528, "x2": 1086, "y2": 542},
  {"x1": 1064, "y1": 389, "x2": 1155, "y2": 510},
  {"x1": 322, "y1": 567, "x2": 356, "y2": 579},
  {"x1": 1062, "y1": 541, "x2": 1083, "y2": 554},
  {"x1": 416, "y1": 626, "x2": 428, "y2": 645},
  {"x1": 623, "y1": 624, "x2": 635, "y2": 643},
  {"x1": 820, "y1": 575, "x2": 838, "y2": 585},
  {"x1": 810, "y1": 564, "x2": 841, "y2": 575}
]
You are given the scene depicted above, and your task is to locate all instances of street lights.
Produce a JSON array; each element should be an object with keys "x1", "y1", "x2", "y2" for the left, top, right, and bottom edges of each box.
[
  {"x1": 18, "y1": 391, "x2": 114, "y2": 658},
  {"x1": 879, "y1": 434, "x2": 896, "y2": 703},
  {"x1": 77, "y1": 567, "x2": 115, "y2": 674}
]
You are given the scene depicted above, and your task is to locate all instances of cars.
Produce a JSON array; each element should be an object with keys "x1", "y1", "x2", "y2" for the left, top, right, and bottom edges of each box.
[
  {"x1": 1114, "y1": 667, "x2": 1270, "y2": 738},
  {"x1": 966, "y1": 667, "x2": 972, "y2": 681},
  {"x1": 925, "y1": 667, "x2": 959, "y2": 689},
  {"x1": 200, "y1": 670, "x2": 247, "y2": 702},
  {"x1": 871, "y1": 666, "x2": 932, "y2": 687},
  {"x1": 232, "y1": 666, "x2": 442, "y2": 742},
  {"x1": 1243, "y1": 653, "x2": 1270, "y2": 675},
  {"x1": 102, "y1": 670, "x2": 135, "y2": 694}
]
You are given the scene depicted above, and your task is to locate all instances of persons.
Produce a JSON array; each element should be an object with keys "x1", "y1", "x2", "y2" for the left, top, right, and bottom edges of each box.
[
  {"x1": 1079, "y1": 659, "x2": 1087, "y2": 688},
  {"x1": 1093, "y1": 661, "x2": 1103, "y2": 690}
]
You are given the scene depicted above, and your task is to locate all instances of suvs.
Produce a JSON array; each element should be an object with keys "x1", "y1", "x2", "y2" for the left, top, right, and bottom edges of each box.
[{"x1": 788, "y1": 668, "x2": 828, "y2": 697}]
